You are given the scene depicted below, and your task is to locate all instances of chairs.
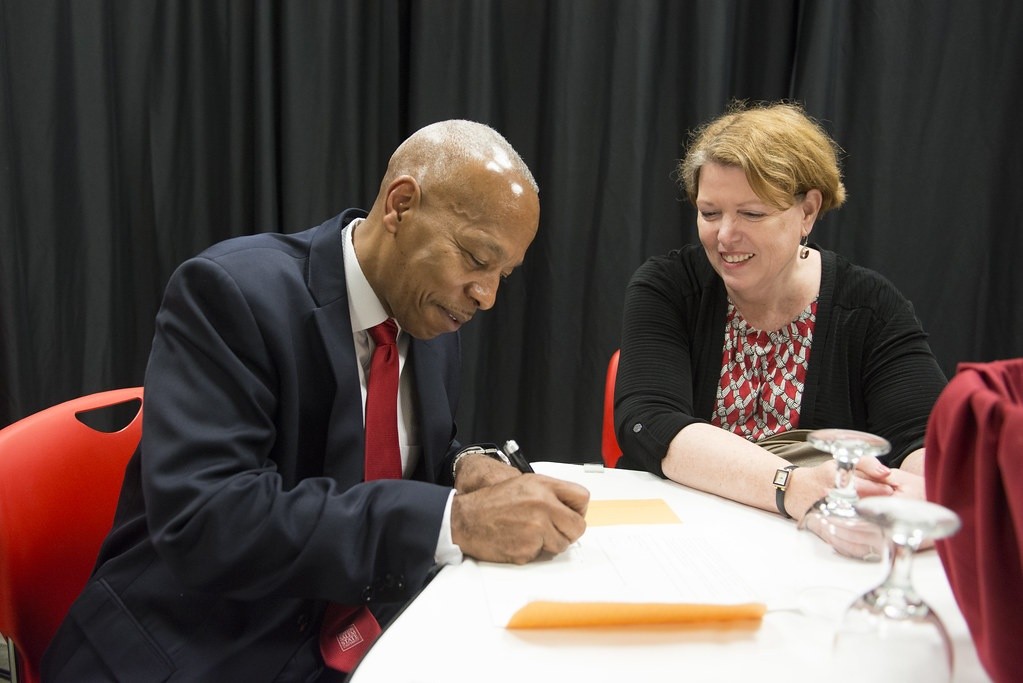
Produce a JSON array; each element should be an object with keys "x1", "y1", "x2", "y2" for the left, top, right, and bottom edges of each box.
[
  {"x1": 0, "y1": 386, "x2": 144, "y2": 683},
  {"x1": 601, "y1": 349, "x2": 623, "y2": 468}
]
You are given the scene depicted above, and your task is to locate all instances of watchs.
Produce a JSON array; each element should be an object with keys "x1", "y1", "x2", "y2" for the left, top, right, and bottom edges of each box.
[
  {"x1": 773, "y1": 466, "x2": 800, "y2": 519},
  {"x1": 452, "y1": 449, "x2": 511, "y2": 480}
]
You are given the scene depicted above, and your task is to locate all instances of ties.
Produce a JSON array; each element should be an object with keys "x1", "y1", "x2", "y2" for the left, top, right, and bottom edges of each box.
[{"x1": 317, "y1": 319, "x2": 406, "y2": 674}]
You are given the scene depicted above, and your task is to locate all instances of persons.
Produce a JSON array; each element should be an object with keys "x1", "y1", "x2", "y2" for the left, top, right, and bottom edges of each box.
[
  {"x1": 613, "y1": 104, "x2": 950, "y2": 561},
  {"x1": 43, "y1": 118, "x2": 589, "y2": 683}
]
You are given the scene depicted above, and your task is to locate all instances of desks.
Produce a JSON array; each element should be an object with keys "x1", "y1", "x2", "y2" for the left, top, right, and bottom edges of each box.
[{"x1": 347, "y1": 461, "x2": 996, "y2": 683}]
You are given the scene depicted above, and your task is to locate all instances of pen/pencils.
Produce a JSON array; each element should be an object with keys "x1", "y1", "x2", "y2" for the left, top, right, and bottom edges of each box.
[{"x1": 503, "y1": 440, "x2": 583, "y2": 549}]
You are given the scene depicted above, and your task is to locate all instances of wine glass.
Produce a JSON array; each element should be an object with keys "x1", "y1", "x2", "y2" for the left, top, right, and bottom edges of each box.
[
  {"x1": 796, "y1": 427, "x2": 892, "y2": 612},
  {"x1": 821, "y1": 493, "x2": 966, "y2": 683}
]
